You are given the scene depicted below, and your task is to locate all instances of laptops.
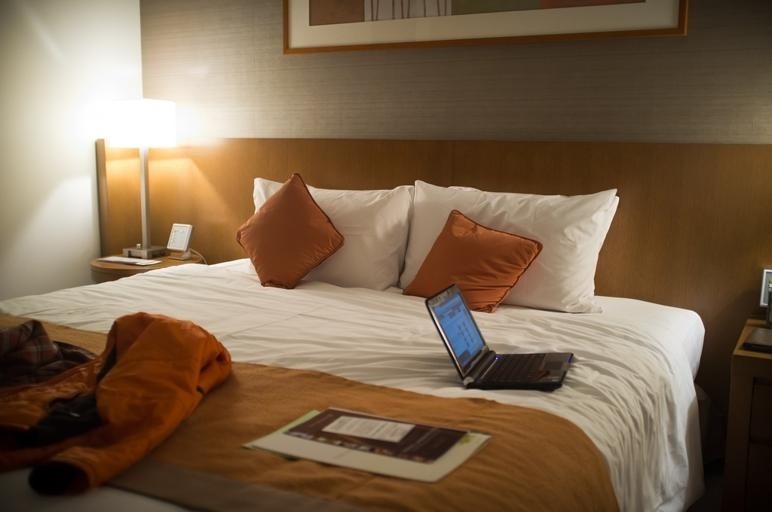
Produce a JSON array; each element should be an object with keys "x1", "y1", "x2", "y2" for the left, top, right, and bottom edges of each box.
[{"x1": 424, "y1": 283, "x2": 573, "y2": 391}]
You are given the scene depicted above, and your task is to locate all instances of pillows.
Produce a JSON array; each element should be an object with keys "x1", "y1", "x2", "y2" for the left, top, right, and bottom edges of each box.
[
  {"x1": 239, "y1": 170, "x2": 345, "y2": 292},
  {"x1": 399, "y1": 178, "x2": 620, "y2": 313},
  {"x1": 405, "y1": 207, "x2": 545, "y2": 314},
  {"x1": 249, "y1": 177, "x2": 414, "y2": 298}
]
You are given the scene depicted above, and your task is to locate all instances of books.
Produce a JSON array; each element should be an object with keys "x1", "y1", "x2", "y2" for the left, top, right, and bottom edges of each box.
[
  {"x1": 240, "y1": 409, "x2": 493, "y2": 486},
  {"x1": 741, "y1": 326, "x2": 772, "y2": 355},
  {"x1": 286, "y1": 408, "x2": 470, "y2": 465}
]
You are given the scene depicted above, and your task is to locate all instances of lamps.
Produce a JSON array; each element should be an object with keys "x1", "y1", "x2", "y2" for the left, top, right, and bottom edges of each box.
[{"x1": 110, "y1": 98, "x2": 181, "y2": 260}]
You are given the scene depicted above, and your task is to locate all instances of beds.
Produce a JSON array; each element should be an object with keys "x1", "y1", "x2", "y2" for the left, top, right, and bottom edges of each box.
[{"x1": 1, "y1": 258, "x2": 715, "y2": 512}]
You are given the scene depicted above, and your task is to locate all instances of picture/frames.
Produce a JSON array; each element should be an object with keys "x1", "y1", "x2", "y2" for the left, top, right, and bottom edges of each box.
[
  {"x1": 166, "y1": 223, "x2": 193, "y2": 251},
  {"x1": 282, "y1": 0, "x2": 688, "y2": 55},
  {"x1": 759, "y1": 269, "x2": 771, "y2": 309}
]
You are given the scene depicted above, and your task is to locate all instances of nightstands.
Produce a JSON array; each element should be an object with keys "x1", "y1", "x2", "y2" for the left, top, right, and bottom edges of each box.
[
  {"x1": 720, "y1": 317, "x2": 772, "y2": 512},
  {"x1": 88, "y1": 254, "x2": 204, "y2": 285}
]
